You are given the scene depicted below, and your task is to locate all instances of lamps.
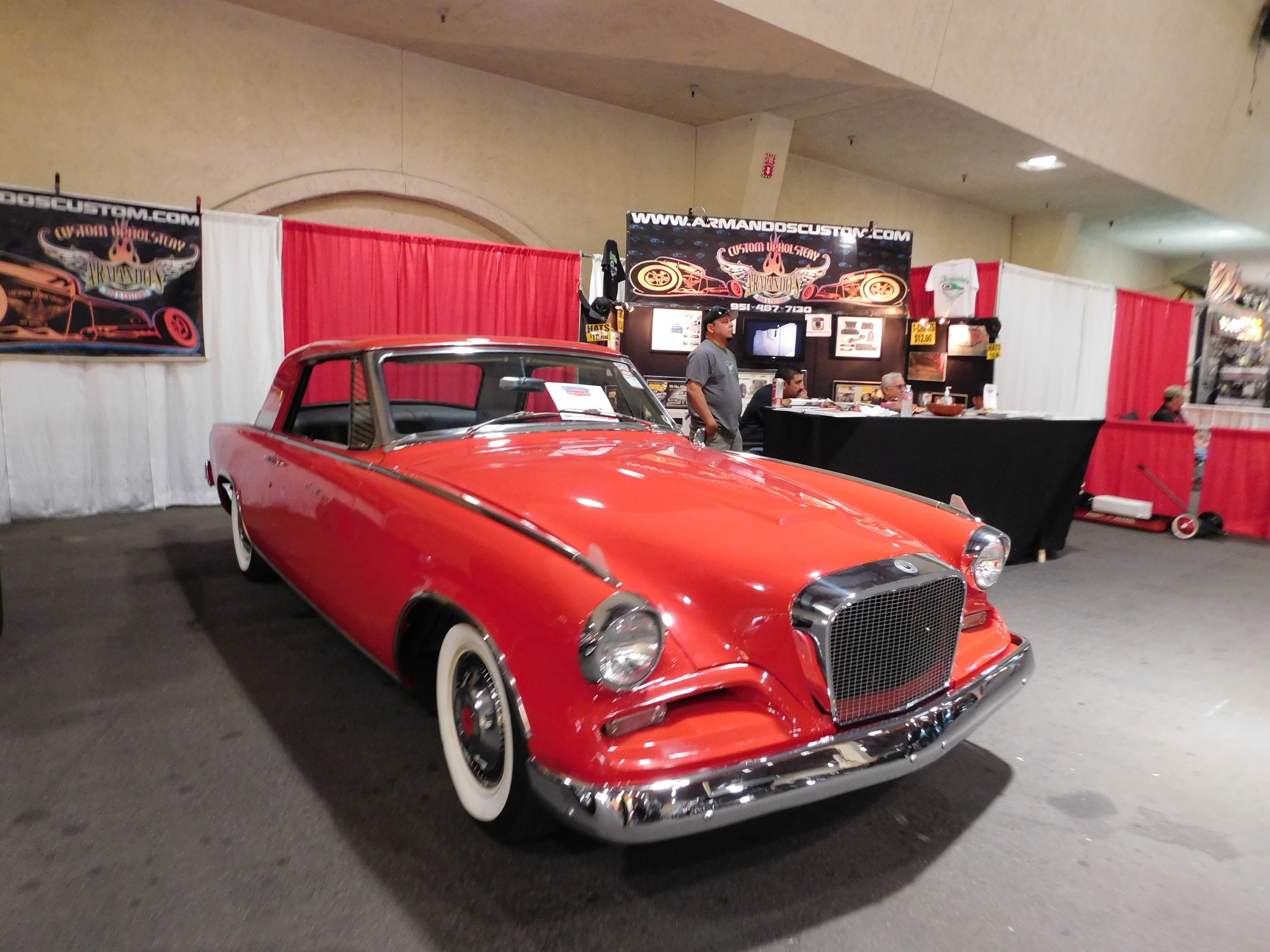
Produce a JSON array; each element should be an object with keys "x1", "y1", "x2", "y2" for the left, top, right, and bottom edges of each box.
[
  {"x1": 864, "y1": 221, "x2": 879, "y2": 237},
  {"x1": 688, "y1": 206, "x2": 710, "y2": 226}
]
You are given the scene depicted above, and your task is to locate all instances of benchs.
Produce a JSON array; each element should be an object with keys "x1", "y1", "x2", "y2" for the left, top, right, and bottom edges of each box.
[{"x1": 294, "y1": 405, "x2": 496, "y2": 444}]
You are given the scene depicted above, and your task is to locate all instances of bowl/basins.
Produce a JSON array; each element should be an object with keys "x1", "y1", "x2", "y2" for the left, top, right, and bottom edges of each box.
[{"x1": 926, "y1": 403, "x2": 966, "y2": 416}]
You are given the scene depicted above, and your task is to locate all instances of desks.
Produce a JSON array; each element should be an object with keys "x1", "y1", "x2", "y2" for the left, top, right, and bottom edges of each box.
[{"x1": 763, "y1": 404, "x2": 1109, "y2": 562}]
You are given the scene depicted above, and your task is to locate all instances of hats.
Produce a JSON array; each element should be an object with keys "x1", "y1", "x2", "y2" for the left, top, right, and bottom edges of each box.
[
  {"x1": 703, "y1": 305, "x2": 739, "y2": 330},
  {"x1": 1163, "y1": 385, "x2": 1191, "y2": 398}
]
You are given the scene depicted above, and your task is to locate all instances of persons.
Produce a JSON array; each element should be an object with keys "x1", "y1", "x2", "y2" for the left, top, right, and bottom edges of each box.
[
  {"x1": 686, "y1": 308, "x2": 742, "y2": 452},
  {"x1": 967, "y1": 383, "x2": 999, "y2": 410},
  {"x1": 870, "y1": 372, "x2": 906, "y2": 405},
  {"x1": 738, "y1": 366, "x2": 812, "y2": 442},
  {"x1": 1151, "y1": 385, "x2": 1192, "y2": 423}
]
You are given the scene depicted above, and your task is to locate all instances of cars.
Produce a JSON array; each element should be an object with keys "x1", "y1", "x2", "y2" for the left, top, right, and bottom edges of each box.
[{"x1": 203, "y1": 332, "x2": 1037, "y2": 850}]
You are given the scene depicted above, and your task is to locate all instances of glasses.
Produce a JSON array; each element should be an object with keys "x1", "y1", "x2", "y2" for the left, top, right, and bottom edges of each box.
[
  {"x1": 896, "y1": 384, "x2": 907, "y2": 389},
  {"x1": 708, "y1": 308, "x2": 727, "y2": 322}
]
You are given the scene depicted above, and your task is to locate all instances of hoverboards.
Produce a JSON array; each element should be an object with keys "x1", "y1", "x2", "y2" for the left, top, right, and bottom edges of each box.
[{"x1": 1171, "y1": 420, "x2": 1224, "y2": 541}]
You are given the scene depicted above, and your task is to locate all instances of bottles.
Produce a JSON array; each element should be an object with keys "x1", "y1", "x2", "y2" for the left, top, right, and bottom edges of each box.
[{"x1": 900, "y1": 385, "x2": 913, "y2": 417}]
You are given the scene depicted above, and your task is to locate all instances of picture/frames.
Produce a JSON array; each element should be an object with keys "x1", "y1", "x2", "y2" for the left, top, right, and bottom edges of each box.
[
  {"x1": 930, "y1": 392, "x2": 968, "y2": 409},
  {"x1": 649, "y1": 305, "x2": 705, "y2": 355},
  {"x1": 643, "y1": 374, "x2": 691, "y2": 436},
  {"x1": 906, "y1": 352, "x2": 947, "y2": 382},
  {"x1": 832, "y1": 380, "x2": 884, "y2": 404},
  {"x1": 736, "y1": 368, "x2": 807, "y2": 416},
  {"x1": 832, "y1": 314, "x2": 886, "y2": 361}
]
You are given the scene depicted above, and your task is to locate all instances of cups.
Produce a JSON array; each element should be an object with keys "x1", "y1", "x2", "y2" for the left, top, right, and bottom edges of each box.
[{"x1": 918, "y1": 391, "x2": 932, "y2": 408}]
